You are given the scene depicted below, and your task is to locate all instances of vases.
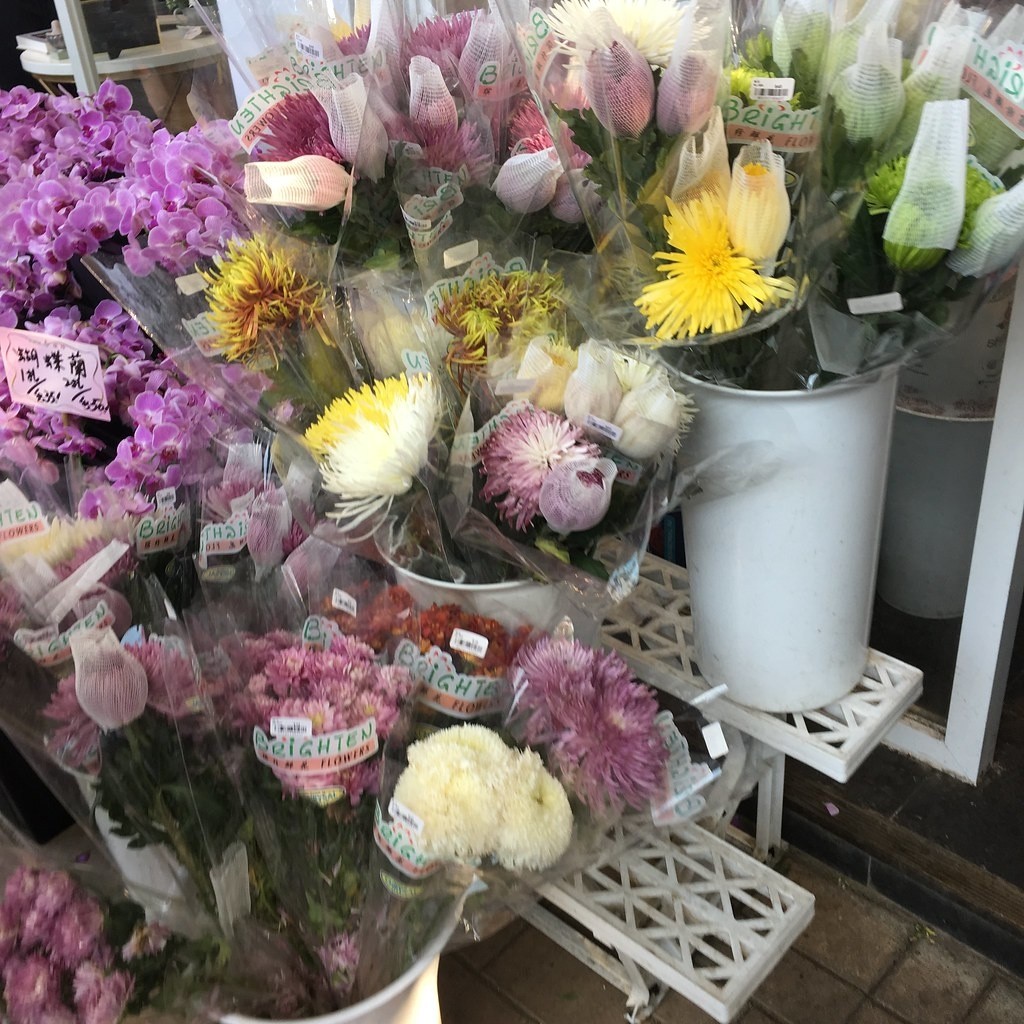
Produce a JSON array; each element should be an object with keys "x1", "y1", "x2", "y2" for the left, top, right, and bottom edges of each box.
[
  {"x1": 651, "y1": 331, "x2": 921, "y2": 713},
  {"x1": 374, "y1": 500, "x2": 571, "y2": 645},
  {"x1": 154, "y1": 855, "x2": 469, "y2": 1024},
  {"x1": 876, "y1": 394, "x2": 996, "y2": 618},
  {"x1": 41, "y1": 733, "x2": 218, "y2": 940}
]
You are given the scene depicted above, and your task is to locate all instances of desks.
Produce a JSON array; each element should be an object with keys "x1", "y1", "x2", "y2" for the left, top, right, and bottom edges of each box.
[{"x1": 19, "y1": 20, "x2": 232, "y2": 118}]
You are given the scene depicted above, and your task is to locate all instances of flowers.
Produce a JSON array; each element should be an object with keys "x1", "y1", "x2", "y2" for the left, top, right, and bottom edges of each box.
[{"x1": 1, "y1": 0, "x2": 1024, "y2": 1024}]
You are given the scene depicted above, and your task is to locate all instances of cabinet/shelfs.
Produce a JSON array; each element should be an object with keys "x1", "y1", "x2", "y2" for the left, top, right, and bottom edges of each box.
[{"x1": 487, "y1": 537, "x2": 923, "y2": 1024}]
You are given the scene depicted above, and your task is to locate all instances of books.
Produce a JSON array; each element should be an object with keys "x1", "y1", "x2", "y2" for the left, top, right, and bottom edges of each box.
[{"x1": 16, "y1": 28, "x2": 68, "y2": 61}]
